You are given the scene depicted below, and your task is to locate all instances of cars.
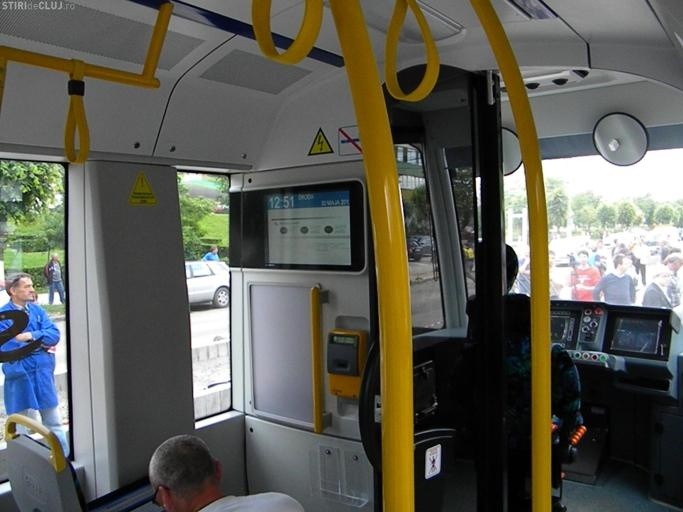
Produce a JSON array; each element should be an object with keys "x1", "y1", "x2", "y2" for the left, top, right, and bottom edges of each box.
[
  {"x1": 405, "y1": 234, "x2": 437, "y2": 262},
  {"x1": 550, "y1": 223, "x2": 682, "y2": 268},
  {"x1": 181, "y1": 256, "x2": 230, "y2": 311}
]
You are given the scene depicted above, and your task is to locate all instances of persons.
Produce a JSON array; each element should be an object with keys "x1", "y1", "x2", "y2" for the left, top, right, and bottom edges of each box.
[
  {"x1": 147, "y1": 433, "x2": 306, "y2": 510},
  {"x1": 201, "y1": 245, "x2": 222, "y2": 262},
  {"x1": 0, "y1": 272, "x2": 60, "y2": 435},
  {"x1": 43, "y1": 251, "x2": 66, "y2": 306},
  {"x1": 460, "y1": 242, "x2": 529, "y2": 334},
  {"x1": 505, "y1": 228, "x2": 682, "y2": 311}
]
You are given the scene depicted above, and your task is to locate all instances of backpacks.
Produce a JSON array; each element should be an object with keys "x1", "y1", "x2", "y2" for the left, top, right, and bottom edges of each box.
[{"x1": 43, "y1": 263, "x2": 50, "y2": 278}]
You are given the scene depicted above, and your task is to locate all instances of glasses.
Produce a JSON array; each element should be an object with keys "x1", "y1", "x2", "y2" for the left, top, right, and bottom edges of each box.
[{"x1": 150, "y1": 483, "x2": 171, "y2": 508}]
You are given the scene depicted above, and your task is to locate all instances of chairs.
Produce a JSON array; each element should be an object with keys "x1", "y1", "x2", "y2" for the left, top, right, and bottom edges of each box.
[{"x1": 4, "y1": 414, "x2": 89, "y2": 512}]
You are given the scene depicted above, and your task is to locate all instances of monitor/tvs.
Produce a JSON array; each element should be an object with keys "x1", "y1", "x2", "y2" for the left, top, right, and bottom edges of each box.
[
  {"x1": 607, "y1": 314, "x2": 665, "y2": 358},
  {"x1": 228, "y1": 178, "x2": 368, "y2": 276}
]
